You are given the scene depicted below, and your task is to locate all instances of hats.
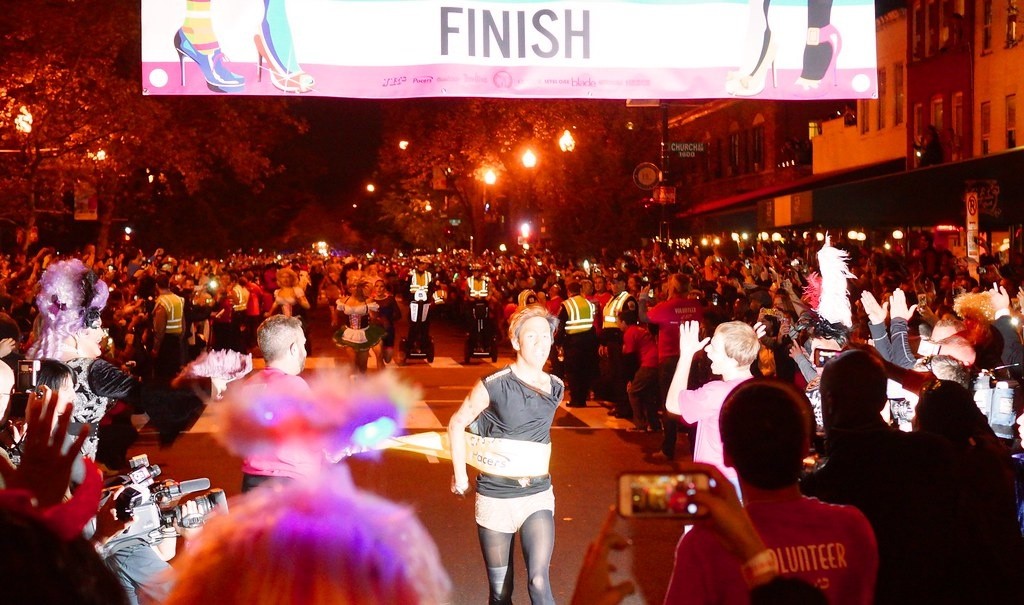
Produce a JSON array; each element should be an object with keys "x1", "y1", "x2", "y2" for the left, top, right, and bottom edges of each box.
[
  {"x1": 750, "y1": 290, "x2": 771, "y2": 305},
  {"x1": 609, "y1": 269, "x2": 627, "y2": 283},
  {"x1": 568, "y1": 282, "x2": 581, "y2": 293},
  {"x1": 469, "y1": 263, "x2": 483, "y2": 272},
  {"x1": 417, "y1": 257, "x2": 432, "y2": 264}
]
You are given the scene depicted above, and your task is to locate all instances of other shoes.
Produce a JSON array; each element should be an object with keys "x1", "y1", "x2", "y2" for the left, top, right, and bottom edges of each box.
[
  {"x1": 590, "y1": 391, "x2": 674, "y2": 459},
  {"x1": 566, "y1": 398, "x2": 587, "y2": 408}
]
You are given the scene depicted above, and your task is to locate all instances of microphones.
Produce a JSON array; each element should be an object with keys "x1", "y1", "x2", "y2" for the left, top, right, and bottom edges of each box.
[{"x1": 103, "y1": 454, "x2": 210, "y2": 497}]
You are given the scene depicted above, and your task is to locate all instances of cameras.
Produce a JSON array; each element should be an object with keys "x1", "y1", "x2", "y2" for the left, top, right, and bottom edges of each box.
[
  {"x1": 8, "y1": 359, "x2": 44, "y2": 422},
  {"x1": 977, "y1": 267, "x2": 988, "y2": 275},
  {"x1": 779, "y1": 281, "x2": 787, "y2": 288},
  {"x1": 648, "y1": 289, "x2": 653, "y2": 299}
]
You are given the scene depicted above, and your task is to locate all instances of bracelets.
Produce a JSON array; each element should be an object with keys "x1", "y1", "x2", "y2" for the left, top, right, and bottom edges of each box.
[{"x1": 741, "y1": 547, "x2": 776, "y2": 578}]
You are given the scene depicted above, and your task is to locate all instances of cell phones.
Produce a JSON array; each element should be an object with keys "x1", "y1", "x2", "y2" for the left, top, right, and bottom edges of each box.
[
  {"x1": 918, "y1": 294, "x2": 926, "y2": 312},
  {"x1": 617, "y1": 470, "x2": 712, "y2": 519}
]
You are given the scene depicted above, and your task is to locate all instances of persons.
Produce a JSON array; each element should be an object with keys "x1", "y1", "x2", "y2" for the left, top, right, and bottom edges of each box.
[
  {"x1": 597, "y1": 267, "x2": 640, "y2": 417},
  {"x1": 0, "y1": 243, "x2": 453, "y2": 605},
  {"x1": 637, "y1": 272, "x2": 707, "y2": 462},
  {"x1": 401, "y1": 254, "x2": 448, "y2": 304},
  {"x1": 664, "y1": 376, "x2": 880, "y2": 605},
  {"x1": 369, "y1": 278, "x2": 402, "y2": 362},
  {"x1": 263, "y1": 265, "x2": 310, "y2": 320},
  {"x1": 237, "y1": 310, "x2": 328, "y2": 496},
  {"x1": 448, "y1": 306, "x2": 564, "y2": 605},
  {"x1": 556, "y1": 281, "x2": 600, "y2": 408},
  {"x1": 569, "y1": 460, "x2": 831, "y2": 605},
  {"x1": 461, "y1": 263, "x2": 492, "y2": 303},
  {"x1": 330, "y1": 274, "x2": 388, "y2": 385},
  {"x1": 449, "y1": 236, "x2": 1024, "y2": 605}
]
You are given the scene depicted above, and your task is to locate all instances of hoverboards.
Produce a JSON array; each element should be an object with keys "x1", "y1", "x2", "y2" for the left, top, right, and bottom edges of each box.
[
  {"x1": 397, "y1": 300, "x2": 432, "y2": 366},
  {"x1": 464, "y1": 303, "x2": 491, "y2": 365}
]
